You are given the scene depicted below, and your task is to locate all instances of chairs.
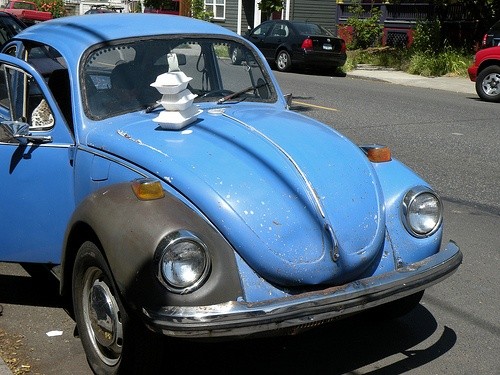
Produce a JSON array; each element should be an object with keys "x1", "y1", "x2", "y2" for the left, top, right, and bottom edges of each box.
[{"x1": 277, "y1": 29, "x2": 286, "y2": 37}]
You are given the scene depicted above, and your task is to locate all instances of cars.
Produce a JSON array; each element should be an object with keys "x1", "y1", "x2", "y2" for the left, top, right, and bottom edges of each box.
[
  {"x1": 85, "y1": 4, "x2": 124, "y2": 14},
  {"x1": 227, "y1": 20, "x2": 347, "y2": 71},
  {"x1": 0, "y1": 13, "x2": 463, "y2": 375},
  {"x1": 468, "y1": 46, "x2": 500, "y2": 103},
  {"x1": 0, "y1": 11, "x2": 67, "y2": 99}
]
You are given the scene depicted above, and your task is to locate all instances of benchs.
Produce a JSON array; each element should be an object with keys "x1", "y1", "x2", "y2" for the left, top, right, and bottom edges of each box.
[{"x1": 31, "y1": 69, "x2": 108, "y2": 130}]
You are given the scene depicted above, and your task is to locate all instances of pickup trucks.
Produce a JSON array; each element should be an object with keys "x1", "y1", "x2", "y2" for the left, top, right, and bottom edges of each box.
[{"x1": 0, "y1": 0, "x2": 52, "y2": 26}]
